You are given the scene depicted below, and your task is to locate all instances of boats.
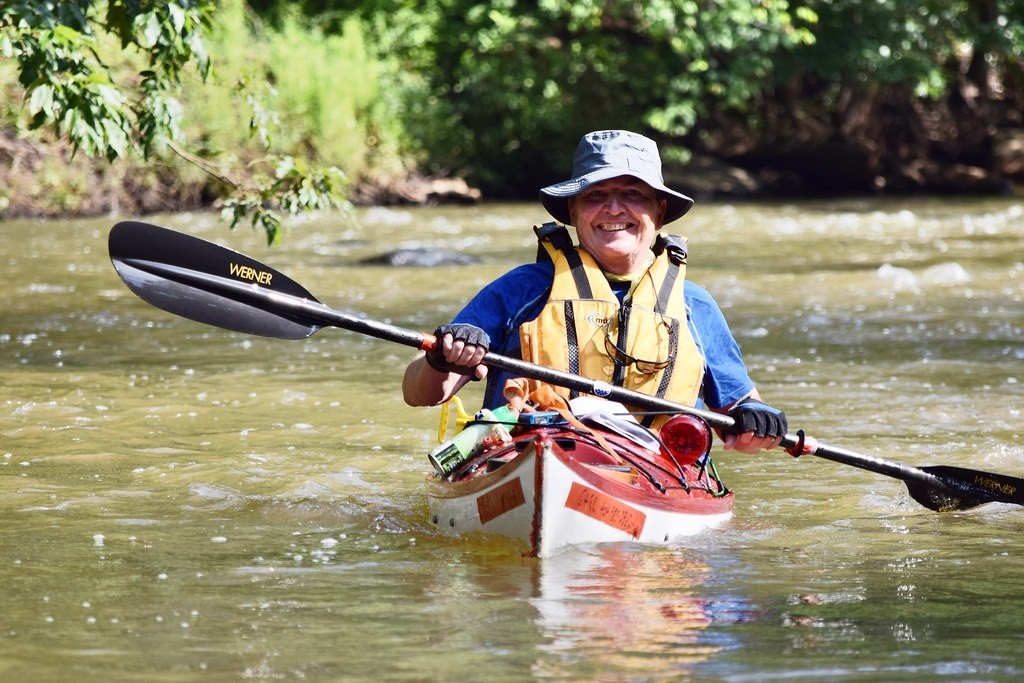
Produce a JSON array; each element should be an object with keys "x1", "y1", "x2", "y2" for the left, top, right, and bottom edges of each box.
[{"x1": 424, "y1": 412, "x2": 736, "y2": 554}]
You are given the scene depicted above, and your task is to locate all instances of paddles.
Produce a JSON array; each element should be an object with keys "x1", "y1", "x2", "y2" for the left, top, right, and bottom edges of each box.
[{"x1": 103, "y1": 216, "x2": 1022, "y2": 518}]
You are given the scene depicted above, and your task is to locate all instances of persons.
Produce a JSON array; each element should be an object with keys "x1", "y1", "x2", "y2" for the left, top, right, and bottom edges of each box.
[{"x1": 402, "y1": 130, "x2": 788, "y2": 455}]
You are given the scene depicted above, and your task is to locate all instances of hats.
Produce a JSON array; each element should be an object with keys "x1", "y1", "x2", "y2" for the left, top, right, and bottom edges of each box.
[{"x1": 540, "y1": 129, "x2": 694, "y2": 225}]
